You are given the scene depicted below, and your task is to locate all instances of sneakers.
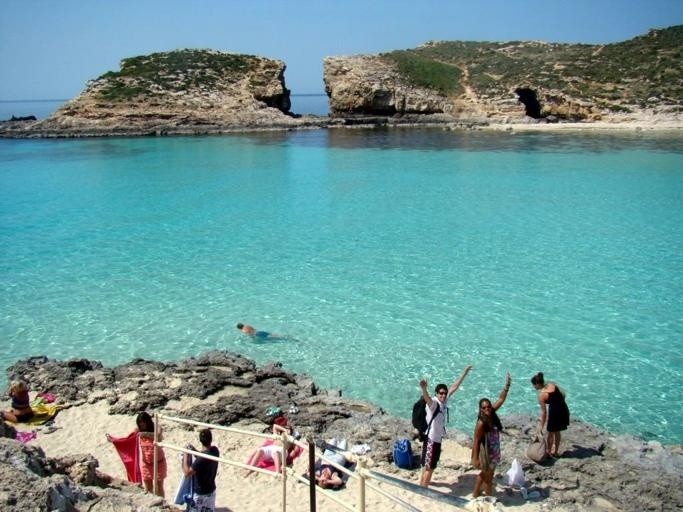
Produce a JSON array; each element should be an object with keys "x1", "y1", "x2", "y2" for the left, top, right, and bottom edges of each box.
[
  {"x1": 521, "y1": 487, "x2": 540, "y2": 500},
  {"x1": 289, "y1": 406, "x2": 299, "y2": 414}
]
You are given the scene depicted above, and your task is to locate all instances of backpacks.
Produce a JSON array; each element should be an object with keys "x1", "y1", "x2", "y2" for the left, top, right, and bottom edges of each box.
[{"x1": 412, "y1": 396, "x2": 439, "y2": 431}]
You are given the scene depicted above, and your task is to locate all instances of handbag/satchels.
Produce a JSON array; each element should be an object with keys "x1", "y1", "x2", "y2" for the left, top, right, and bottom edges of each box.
[
  {"x1": 471, "y1": 443, "x2": 489, "y2": 470},
  {"x1": 394, "y1": 440, "x2": 412, "y2": 467},
  {"x1": 527, "y1": 433, "x2": 545, "y2": 462}
]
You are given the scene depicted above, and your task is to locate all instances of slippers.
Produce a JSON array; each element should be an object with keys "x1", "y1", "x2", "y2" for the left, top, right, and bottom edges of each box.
[
  {"x1": 266, "y1": 407, "x2": 281, "y2": 416},
  {"x1": 43, "y1": 421, "x2": 62, "y2": 434}
]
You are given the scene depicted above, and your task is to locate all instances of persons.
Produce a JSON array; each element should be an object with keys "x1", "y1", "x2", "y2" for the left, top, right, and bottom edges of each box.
[
  {"x1": 307, "y1": 438, "x2": 346, "y2": 489},
  {"x1": 471, "y1": 371, "x2": 511, "y2": 498},
  {"x1": 237, "y1": 324, "x2": 284, "y2": 340},
  {"x1": 107, "y1": 412, "x2": 167, "y2": 497},
  {"x1": 182, "y1": 429, "x2": 219, "y2": 512},
  {"x1": 0, "y1": 379, "x2": 35, "y2": 422},
  {"x1": 420, "y1": 365, "x2": 472, "y2": 486},
  {"x1": 532, "y1": 373, "x2": 569, "y2": 456},
  {"x1": 243, "y1": 424, "x2": 292, "y2": 481}
]
